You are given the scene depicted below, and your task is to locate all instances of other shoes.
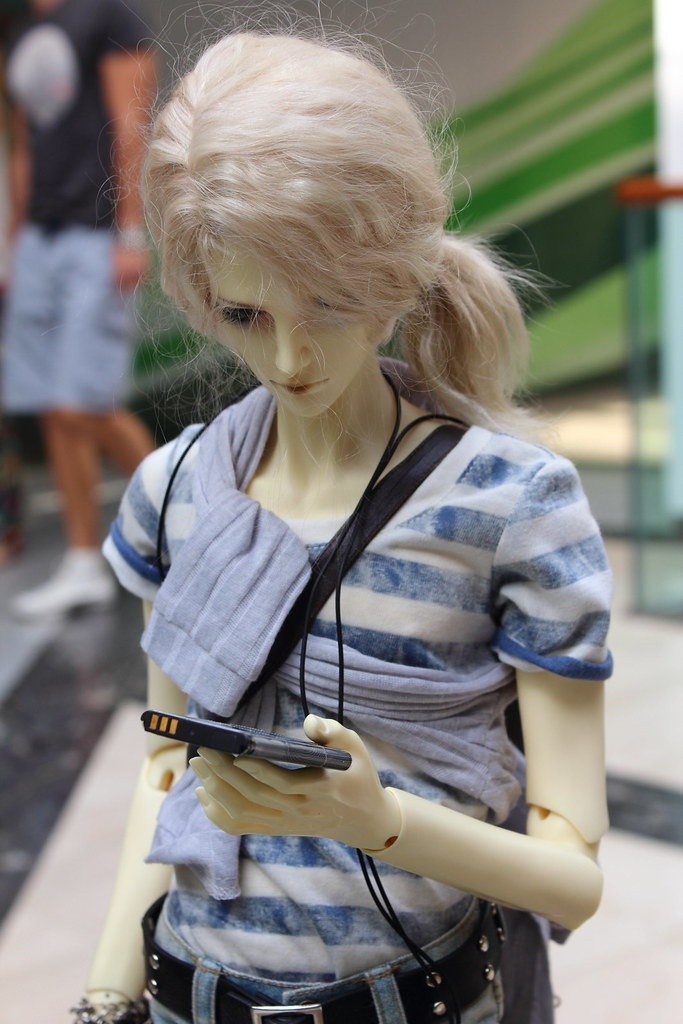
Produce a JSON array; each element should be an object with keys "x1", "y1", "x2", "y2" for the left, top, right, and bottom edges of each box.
[{"x1": 11, "y1": 548, "x2": 115, "y2": 617}]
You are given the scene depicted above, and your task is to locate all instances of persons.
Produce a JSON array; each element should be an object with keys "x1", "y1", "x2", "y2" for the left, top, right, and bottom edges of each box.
[
  {"x1": 64, "y1": 0, "x2": 615, "y2": 1024},
  {"x1": 0, "y1": 0, "x2": 158, "y2": 621}
]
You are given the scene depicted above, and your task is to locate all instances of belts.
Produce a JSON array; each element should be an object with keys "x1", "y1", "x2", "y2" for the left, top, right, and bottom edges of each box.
[{"x1": 141, "y1": 891, "x2": 506, "y2": 1024}]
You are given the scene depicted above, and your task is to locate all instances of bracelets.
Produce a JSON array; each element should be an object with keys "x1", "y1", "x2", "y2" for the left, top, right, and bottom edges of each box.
[
  {"x1": 67, "y1": 993, "x2": 151, "y2": 1024},
  {"x1": 113, "y1": 227, "x2": 149, "y2": 250}
]
